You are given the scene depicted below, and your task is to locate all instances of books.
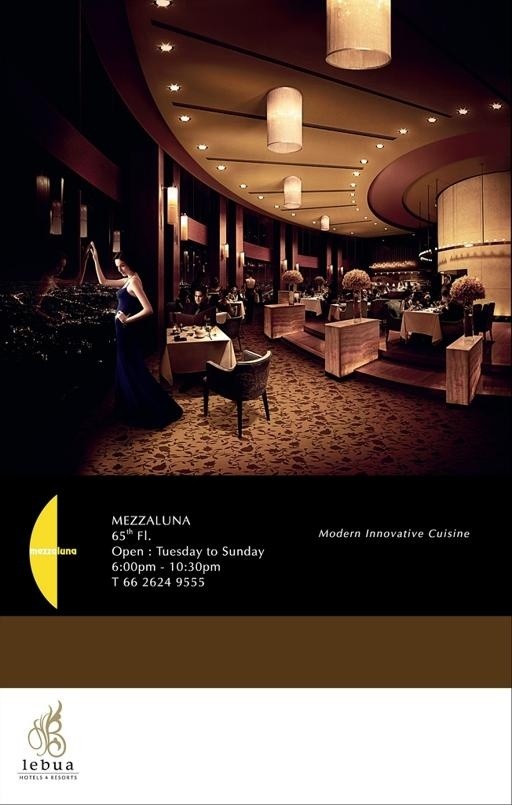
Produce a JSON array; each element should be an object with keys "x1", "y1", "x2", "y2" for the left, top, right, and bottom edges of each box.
[{"x1": 174, "y1": 305, "x2": 217, "y2": 326}]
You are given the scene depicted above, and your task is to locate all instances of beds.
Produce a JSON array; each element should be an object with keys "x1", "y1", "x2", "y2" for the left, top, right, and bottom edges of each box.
[{"x1": 160, "y1": 290, "x2": 464, "y2": 386}]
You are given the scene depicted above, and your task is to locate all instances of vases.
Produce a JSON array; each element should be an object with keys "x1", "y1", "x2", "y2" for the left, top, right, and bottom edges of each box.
[
  {"x1": 463, "y1": 301, "x2": 473, "y2": 337},
  {"x1": 288, "y1": 284, "x2": 295, "y2": 305}
]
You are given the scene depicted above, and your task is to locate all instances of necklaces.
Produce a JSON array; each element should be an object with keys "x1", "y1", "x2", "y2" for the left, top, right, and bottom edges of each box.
[{"x1": 128, "y1": 272, "x2": 135, "y2": 279}]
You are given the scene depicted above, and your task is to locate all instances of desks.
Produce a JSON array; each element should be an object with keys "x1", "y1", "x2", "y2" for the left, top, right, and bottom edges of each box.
[{"x1": 445, "y1": 333, "x2": 483, "y2": 406}]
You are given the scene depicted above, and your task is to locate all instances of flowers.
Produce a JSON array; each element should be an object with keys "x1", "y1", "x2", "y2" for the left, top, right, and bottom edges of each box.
[
  {"x1": 341, "y1": 268, "x2": 371, "y2": 289},
  {"x1": 449, "y1": 275, "x2": 485, "y2": 301},
  {"x1": 280, "y1": 270, "x2": 303, "y2": 283},
  {"x1": 314, "y1": 276, "x2": 325, "y2": 286}
]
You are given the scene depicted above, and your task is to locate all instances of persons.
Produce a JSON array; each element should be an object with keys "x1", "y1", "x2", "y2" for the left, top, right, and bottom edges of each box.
[
  {"x1": 243, "y1": 269, "x2": 256, "y2": 323},
  {"x1": 174, "y1": 276, "x2": 242, "y2": 316},
  {"x1": 89, "y1": 240, "x2": 184, "y2": 430},
  {"x1": 361, "y1": 274, "x2": 465, "y2": 344},
  {"x1": 172, "y1": 287, "x2": 216, "y2": 327},
  {"x1": 319, "y1": 284, "x2": 336, "y2": 319}
]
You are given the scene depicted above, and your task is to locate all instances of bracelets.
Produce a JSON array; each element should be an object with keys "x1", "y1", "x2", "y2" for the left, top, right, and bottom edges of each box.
[{"x1": 124, "y1": 317, "x2": 127, "y2": 325}]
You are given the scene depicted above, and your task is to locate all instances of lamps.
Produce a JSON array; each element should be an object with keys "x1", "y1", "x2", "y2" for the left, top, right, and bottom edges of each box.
[
  {"x1": 281, "y1": 260, "x2": 287, "y2": 269},
  {"x1": 295, "y1": 263, "x2": 299, "y2": 271},
  {"x1": 180, "y1": 213, "x2": 188, "y2": 241},
  {"x1": 283, "y1": 175, "x2": 302, "y2": 209},
  {"x1": 49, "y1": 200, "x2": 62, "y2": 236},
  {"x1": 112, "y1": 230, "x2": 121, "y2": 253},
  {"x1": 320, "y1": 215, "x2": 329, "y2": 231},
  {"x1": 328, "y1": 265, "x2": 333, "y2": 274},
  {"x1": 80, "y1": 203, "x2": 88, "y2": 238},
  {"x1": 325, "y1": 0, "x2": 392, "y2": 70},
  {"x1": 161, "y1": 182, "x2": 179, "y2": 224},
  {"x1": 340, "y1": 267, "x2": 343, "y2": 275},
  {"x1": 36, "y1": 175, "x2": 51, "y2": 202},
  {"x1": 222, "y1": 243, "x2": 229, "y2": 258},
  {"x1": 239, "y1": 252, "x2": 245, "y2": 264},
  {"x1": 266, "y1": 86, "x2": 303, "y2": 154}
]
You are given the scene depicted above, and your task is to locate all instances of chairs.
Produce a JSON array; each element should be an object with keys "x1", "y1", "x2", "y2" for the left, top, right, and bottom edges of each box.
[
  {"x1": 202, "y1": 350, "x2": 272, "y2": 437},
  {"x1": 473, "y1": 303, "x2": 495, "y2": 340}
]
